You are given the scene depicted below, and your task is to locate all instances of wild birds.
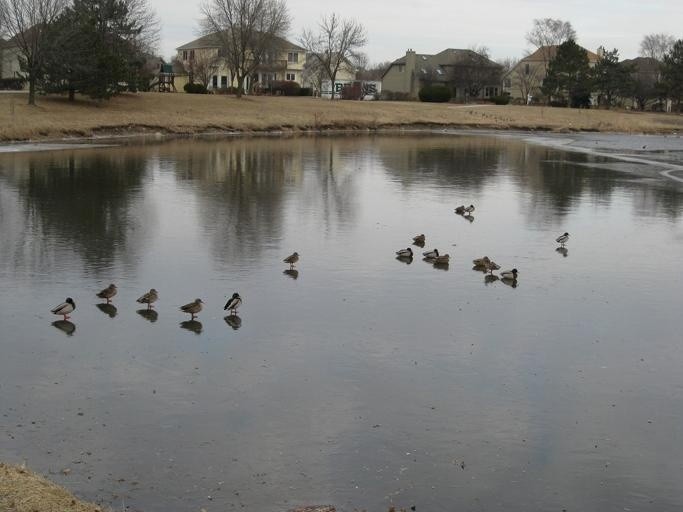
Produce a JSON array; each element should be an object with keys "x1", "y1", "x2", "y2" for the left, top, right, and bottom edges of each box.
[
  {"x1": 555, "y1": 246, "x2": 568, "y2": 257},
  {"x1": 472, "y1": 256, "x2": 520, "y2": 278},
  {"x1": 51, "y1": 301, "x2": 242, "y2": 336},
  {"x1": 455, "y1": 204, "x2": 475, "y2": 215},
  {"x1": 51, "y1": 283, "x2": 244, "y2": 319},
  {"x1": 395, "y1": 234, "x2": 449, "y2": 264},
  {"x1": 555, "y1": 233, "x2": 569, "y2": 245},
  {"x1": 283, "y1": 252, "x2": 300, "y2": 266},
  {"x1": 283, "y1": 266, "x2": 299, "y2": 280}
]
